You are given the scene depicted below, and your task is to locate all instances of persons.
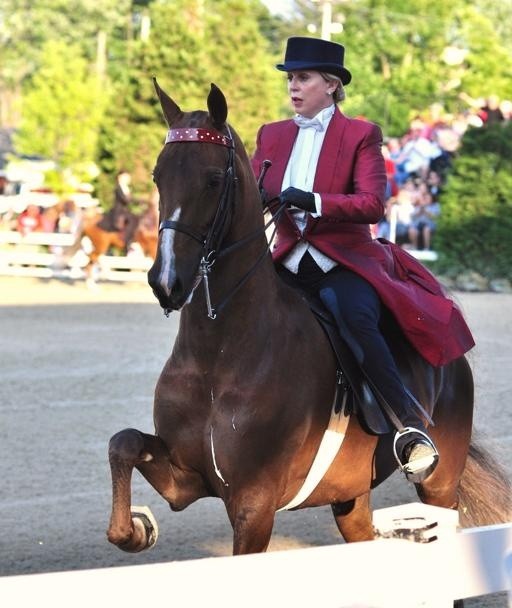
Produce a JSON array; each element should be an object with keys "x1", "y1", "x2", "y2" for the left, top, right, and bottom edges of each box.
[
  {"x1": 109, "y1": 167, "x2": 149, "y2": 253},
  {"x1": 369, "y1": 90, "x2": 511, "y2": 252},
  {"x1": 244, "y1": 34, "x2": 440, "y2": 485}
]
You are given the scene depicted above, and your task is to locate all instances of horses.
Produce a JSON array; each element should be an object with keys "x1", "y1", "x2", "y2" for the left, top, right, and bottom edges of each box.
[{"x1": 106, "y1": 78, "x2": 512, "y2": 560}]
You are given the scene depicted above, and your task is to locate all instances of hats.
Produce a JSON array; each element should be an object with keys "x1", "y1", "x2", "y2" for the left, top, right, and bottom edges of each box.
[{"x1": 275, "y1": 36, "x2": 352, "y2": 85}]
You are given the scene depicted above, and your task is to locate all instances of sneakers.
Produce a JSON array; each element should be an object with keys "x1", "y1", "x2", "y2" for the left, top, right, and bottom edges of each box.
[{"x1": 402, "y1": 420, "x2": 436, "y2": 473}]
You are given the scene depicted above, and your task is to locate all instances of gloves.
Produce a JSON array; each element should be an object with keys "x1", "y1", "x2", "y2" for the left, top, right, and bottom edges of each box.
[{"x1": 279, "y1": 186, "x2": 317, "y2": 214}]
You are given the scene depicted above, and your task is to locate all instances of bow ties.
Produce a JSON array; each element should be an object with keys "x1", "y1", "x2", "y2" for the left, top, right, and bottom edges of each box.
[{"x1": 292, "y1": 115, "x2": 324, "y2": 132}]
